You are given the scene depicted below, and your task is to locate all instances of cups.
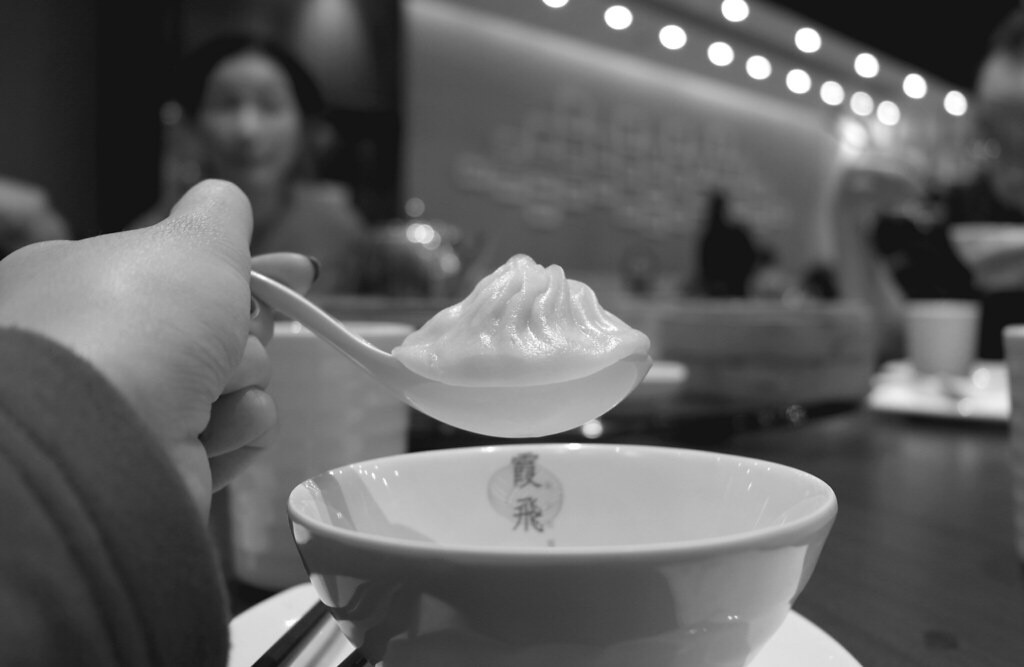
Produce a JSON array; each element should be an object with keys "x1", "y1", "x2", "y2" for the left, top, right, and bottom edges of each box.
[
  {"x1": 217, "y1": 321, "x2": 410, "y2": 585},
  {"x1": 905, "y1": 305, "x2": 979, "y2": 376},
  {"x1": 999, "y1": 327, "x2": 1024, "y2": 408}
]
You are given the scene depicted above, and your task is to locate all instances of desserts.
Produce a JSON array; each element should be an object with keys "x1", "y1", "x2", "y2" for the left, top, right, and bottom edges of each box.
[{"x1": 388, "y1": 252, "x2": 650, "y2": 384}]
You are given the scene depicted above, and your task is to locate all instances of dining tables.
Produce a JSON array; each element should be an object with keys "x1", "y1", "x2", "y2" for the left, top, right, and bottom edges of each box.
[{"x1": 740, "y1": 407, "x2": 1024, "y2": 667}]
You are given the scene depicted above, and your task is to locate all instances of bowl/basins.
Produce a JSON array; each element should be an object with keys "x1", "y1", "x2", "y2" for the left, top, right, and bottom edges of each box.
[{"x1": 286, "y1": 442, "x2": 840, "y2": 667}]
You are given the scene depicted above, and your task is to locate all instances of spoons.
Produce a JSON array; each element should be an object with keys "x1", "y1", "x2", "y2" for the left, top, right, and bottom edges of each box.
[{"x1": 247, "y1": 266, "x2": 652, "y2": 440}]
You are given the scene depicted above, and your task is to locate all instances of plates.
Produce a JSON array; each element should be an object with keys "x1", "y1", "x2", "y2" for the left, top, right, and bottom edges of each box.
[
  {"x1": 863, "y1": 361, "x2": 1012, "y2": 424},
  {"x1": 224, "y1": 579, "x2": 865, "y2": 667}
]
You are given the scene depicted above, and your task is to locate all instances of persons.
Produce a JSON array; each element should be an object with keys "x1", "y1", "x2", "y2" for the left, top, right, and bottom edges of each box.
[
  {"x1": 0, "y1": 179, "x2": 314, "y2": 667},
  {"x1": 693, "y1": 170, "x2": 1024, "y2": 360},
  {"x1": 133, "y1": 40, "x2": 361, "y2": 298}
]
